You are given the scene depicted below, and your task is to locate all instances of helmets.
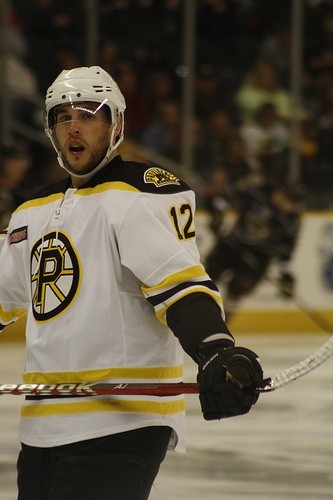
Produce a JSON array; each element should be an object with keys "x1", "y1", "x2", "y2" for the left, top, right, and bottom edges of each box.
[{"x1": 42, "y1": 64, "x2": 126, "y2": 179}]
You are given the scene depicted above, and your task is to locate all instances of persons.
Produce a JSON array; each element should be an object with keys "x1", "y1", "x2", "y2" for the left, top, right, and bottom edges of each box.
[
  {"x1": 0, "y1": 65, "x2": 263, "y2": 500},
  {"x1": 98, "y1": 0, "x2": 333, "y2": 198},
  {"x1": 202, "y1": 180, "x2": 306, "y2": 326},
  {"x1": 1, "y1": 0, "x2": 85, "y2": 228}
]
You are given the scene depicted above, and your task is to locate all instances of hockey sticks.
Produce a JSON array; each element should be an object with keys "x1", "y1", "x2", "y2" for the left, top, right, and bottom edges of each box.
[
  {"x1": 0, "y1": 335, "x2": 333, "y2": 395},
  {"x1": 264, "y1": 275, "x2": 332, "y2": 336}
]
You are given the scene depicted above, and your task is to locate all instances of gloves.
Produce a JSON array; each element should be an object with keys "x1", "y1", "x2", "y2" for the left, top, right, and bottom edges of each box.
[{"x1": 194, "y1": 345, "x2": 264, "y2": 421}]
